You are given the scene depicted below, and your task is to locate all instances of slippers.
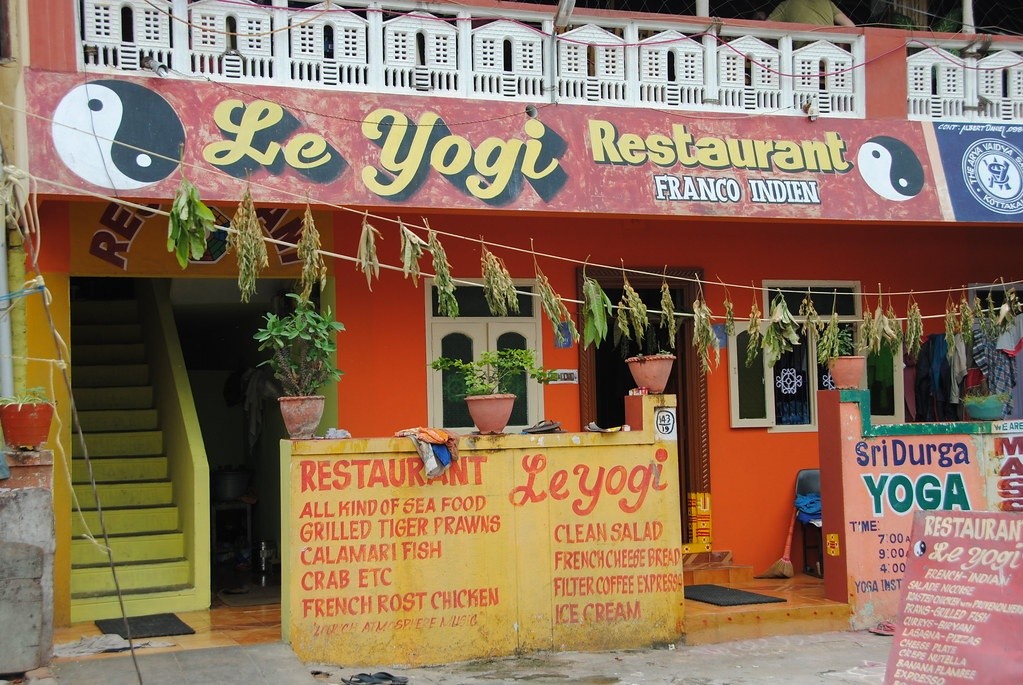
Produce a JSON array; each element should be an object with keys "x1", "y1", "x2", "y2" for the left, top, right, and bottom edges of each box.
[{"x1": 522, "y1": 419, "x2": 568, "y2": 433}]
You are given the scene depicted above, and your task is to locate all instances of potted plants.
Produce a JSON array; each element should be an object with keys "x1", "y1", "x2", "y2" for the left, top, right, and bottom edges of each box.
[
  {"x1": 823, "y1": 324, "x2": 868, "y2": 389},
  {"x1": 424, "y1": 348, "x2": 561, "y2": 436},
  {"x1": 956, "y1": 391, "x2": 1012, "y2": 421},
  {"x1": 1, "y1": 381, "x2": 56, "y2": 449},
  {"x1": 252, "y1": 292, "x2": 347, "y2": 441},
  {"x1": 624, "y1": 341, "x2": 679, "y2": 394}
]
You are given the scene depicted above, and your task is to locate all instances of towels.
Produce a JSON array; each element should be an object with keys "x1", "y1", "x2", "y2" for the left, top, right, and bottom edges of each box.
[{"x1": 394, "y1": 421, "x2": 461, "y2": 482}]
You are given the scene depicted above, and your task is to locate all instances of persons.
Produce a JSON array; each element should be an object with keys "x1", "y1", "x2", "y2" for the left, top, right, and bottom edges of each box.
[{"x1": 766, "y1": 1, "x2": 854, "y2": 56}]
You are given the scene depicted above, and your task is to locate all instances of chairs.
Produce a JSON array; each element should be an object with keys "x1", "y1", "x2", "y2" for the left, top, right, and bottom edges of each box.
[{"x1": 792, "y1": 466, "x2": 825, "y2": 580}]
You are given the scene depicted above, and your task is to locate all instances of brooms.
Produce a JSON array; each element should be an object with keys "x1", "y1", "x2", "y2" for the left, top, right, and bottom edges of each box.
[{"x1": 754, "y1": 497, "x2": 801, "y2": 580}]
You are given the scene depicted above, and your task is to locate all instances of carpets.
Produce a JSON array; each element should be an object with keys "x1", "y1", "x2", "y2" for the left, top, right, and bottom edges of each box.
[
  {"x1": 94, "y1": 612, "x2": 196, "y2": 640},
  {"x1": 684, "y1": 583, "x2": 788, "y2": 607}
]
